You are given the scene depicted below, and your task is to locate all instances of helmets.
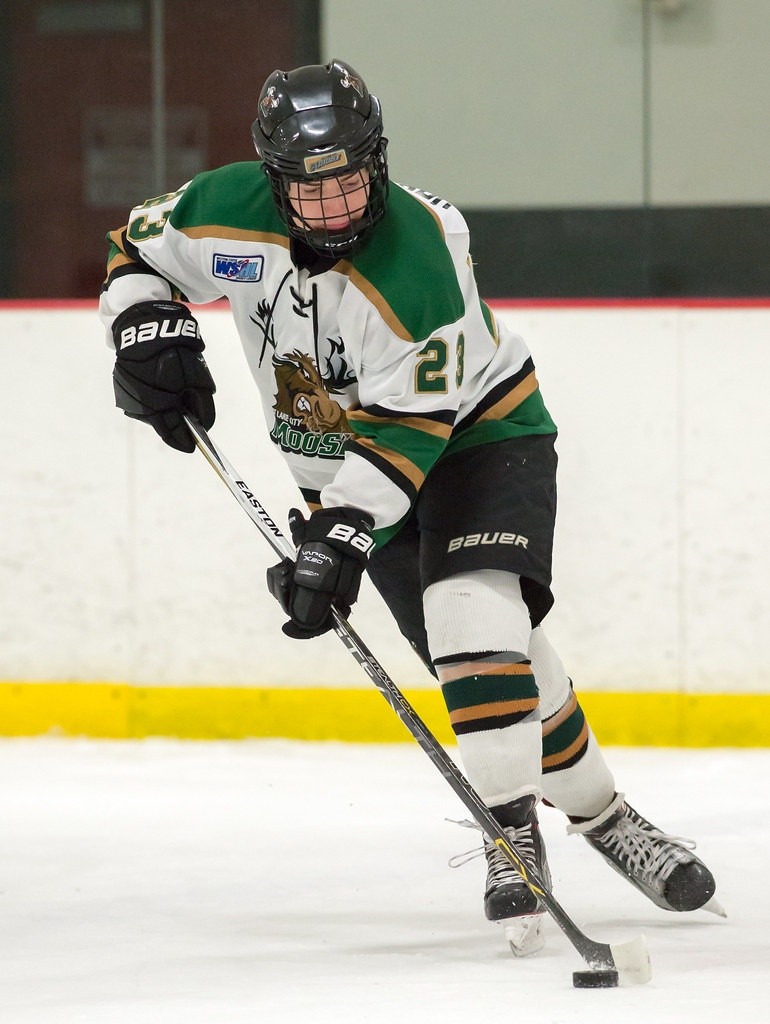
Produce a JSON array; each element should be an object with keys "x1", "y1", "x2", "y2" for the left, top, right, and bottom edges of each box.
[{"x1": 247, "y1": 58, "x2": 385, "y2": 180}]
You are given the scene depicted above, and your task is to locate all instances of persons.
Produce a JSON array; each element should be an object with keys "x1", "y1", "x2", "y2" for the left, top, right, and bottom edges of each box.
[{"x1": 100, "y1": 60, "x2": 728, "y2": 956}]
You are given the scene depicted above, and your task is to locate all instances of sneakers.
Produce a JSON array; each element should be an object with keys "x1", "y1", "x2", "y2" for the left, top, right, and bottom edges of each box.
[
  {"x1": 445, "y1": 783, "x2": 552, "y2": 954},
  {"x1": 542, "y1": 786, "x2": 729, "y2": 919}
]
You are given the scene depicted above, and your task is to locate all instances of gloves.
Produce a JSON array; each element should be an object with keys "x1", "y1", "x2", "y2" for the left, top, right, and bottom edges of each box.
[
  {"x1": 268, "y1": 505, "x2": 374, "y2": 640},
  {"x1": 111, "y1": 300, "x2": 216, "y2": 454}
]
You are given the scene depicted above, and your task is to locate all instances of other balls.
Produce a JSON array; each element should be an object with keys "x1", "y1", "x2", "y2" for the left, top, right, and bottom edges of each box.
[{"x1": 573, "y1": 970, "x2": 619, "y2": 988}]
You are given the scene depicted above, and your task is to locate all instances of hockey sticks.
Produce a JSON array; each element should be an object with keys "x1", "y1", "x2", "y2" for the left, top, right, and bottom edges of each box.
[{"x1": 176, "y1": 412, "x2": 654, "y2": 991}]
